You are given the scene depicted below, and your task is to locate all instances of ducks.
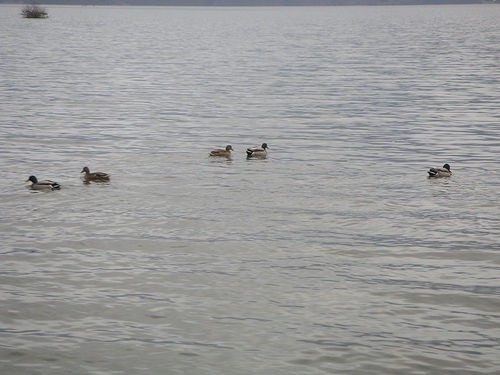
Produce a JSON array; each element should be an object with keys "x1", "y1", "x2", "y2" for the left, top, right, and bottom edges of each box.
[
  {"x1": 246, "y1": 143, "x2": 270, "y2": 156},
  {"x1": 427, "y1": 164, "x2": 452, "y2": 176},
  {"x1": 25, "y1": 176, "x2": 60, "y2": 190},
  {"x1": 210, "y1": 144, "x2": 234, "y2": 156},
  {"x1": 81, "y1": 167, "x2": 110, "y2": 181}
]
